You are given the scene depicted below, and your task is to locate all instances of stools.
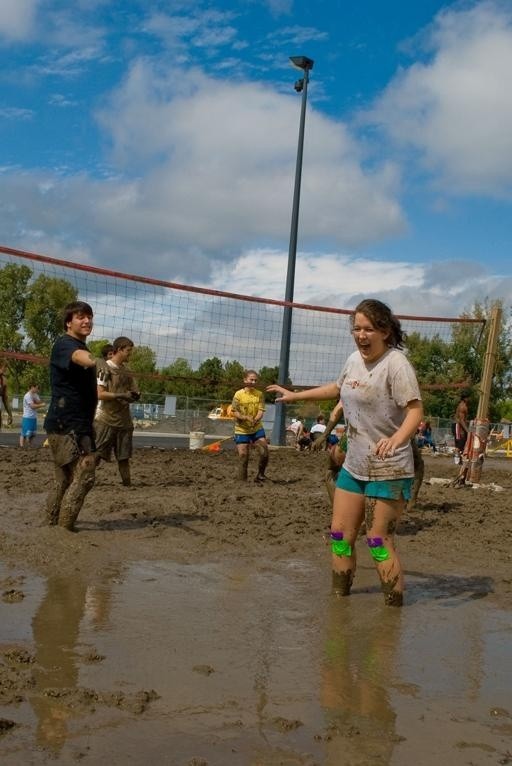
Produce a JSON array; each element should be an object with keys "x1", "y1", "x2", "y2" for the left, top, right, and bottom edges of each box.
[{"x1": 438, "y1": 440, "x2": 447, "y2": 454}]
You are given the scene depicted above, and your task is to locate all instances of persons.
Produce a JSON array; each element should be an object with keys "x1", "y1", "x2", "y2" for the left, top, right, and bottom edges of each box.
[
  {"x1": 452, "y1": 387, "x2": 474, "y2": 465},
  {"x1": 229, "y1": 370, "x2": 269, "y2": 483},
  {"x1": 90, "y1": 336, "x2": 142, "y2": 484},
  {"x1": 101, "y1": 344, "x2": 113, "y2": 361},
  {"x1": 414, "y1": 418, "x2": 439, "y2": 454},
  {"x1": 18, "y1": 380, "x2": 47, "y2": 448},
  {"x1": 264, "y1": 298, "x2": 425, "y2": 607},
  {"x1": 42, "y1": 299, "x2": 118, "y2": 537},
  {"x1": 286, "y1": 415, "x2": 304, "y2": 449},
  {"x1": 310, "y1": 415, "x2": 327, "y2": 451},
  {"x1": 311, "y1": 398, "x2": 424, "y2": 535}
]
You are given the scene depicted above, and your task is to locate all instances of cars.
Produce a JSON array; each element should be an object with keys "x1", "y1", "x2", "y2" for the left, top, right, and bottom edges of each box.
[{"x1": 490, "y1": 431, "x2": 503, "y2": 443}]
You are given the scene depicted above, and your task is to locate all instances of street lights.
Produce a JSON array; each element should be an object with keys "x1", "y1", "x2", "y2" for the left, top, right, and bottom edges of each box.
[{"x1": 270, "y1": 56, "x2": 313, "y2": 446}]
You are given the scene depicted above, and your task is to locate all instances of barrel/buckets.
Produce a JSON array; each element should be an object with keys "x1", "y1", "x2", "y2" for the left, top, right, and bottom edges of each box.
[{"x1": 189, "y1": 432, "x2": 204, "y2": 450}]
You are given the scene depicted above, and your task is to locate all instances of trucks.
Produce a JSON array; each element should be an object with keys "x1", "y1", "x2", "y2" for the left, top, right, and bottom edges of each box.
[{"x1": 207, "y1": 405, "x2": 232, "y2": 419}]
